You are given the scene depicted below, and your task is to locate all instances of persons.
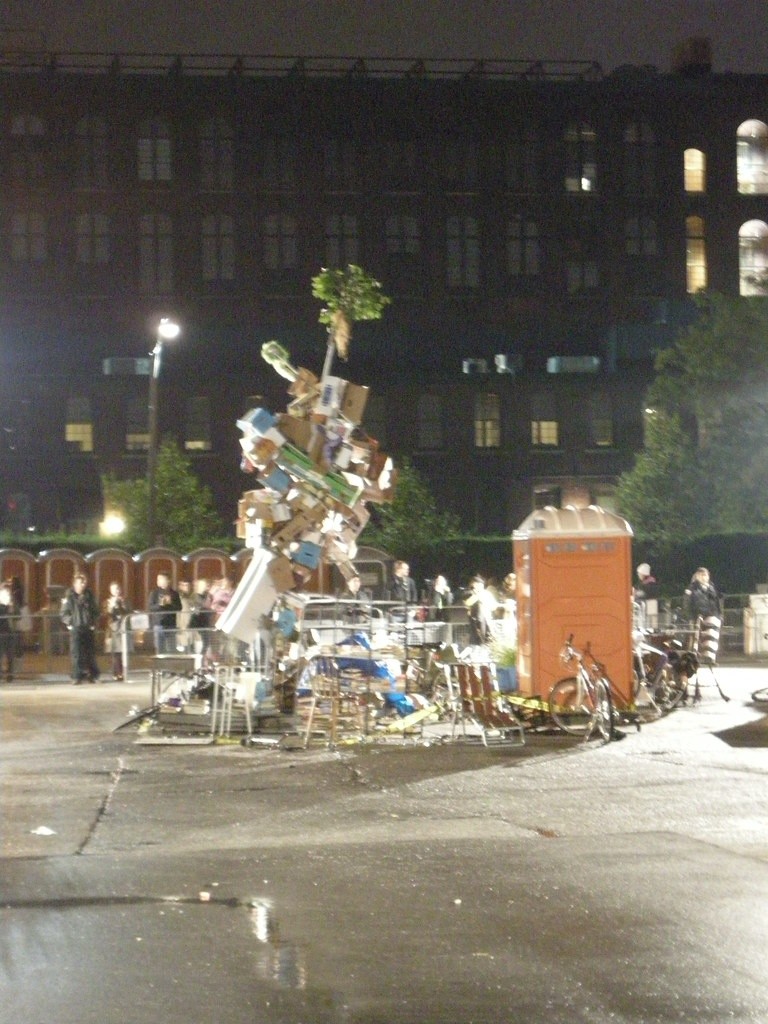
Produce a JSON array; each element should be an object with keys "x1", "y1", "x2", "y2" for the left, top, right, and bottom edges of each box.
[
  {"x1": 175, "y1": 577, "x2": 240, "y2": 668},
  {"x1": 387, "y1": 560, "x2": 419, "y2": 622},
  {"x1": 633, "y1": 563, "x2": 659, "y2": 628},
  {"x1": 429, "y1": 574, "x2": 454, "y2": 622},
  {"x1": 146, "y1": 573, "x2": 183, "y2": 680},
  {"x1": 61, "y1": 574, "x2": 99, "y2": 685},
  {"x1": 457, "y1": 572, "x2": 517, "y2": 695},
  {"x1": 681, "y1": 568, "x2": 725, "y2": 667},
  {"x1": 0, "y1": 575, "x2": 25, "y2": 685},
  {"x1": 102, "y1": 579, "x2": 129, "y2": 682}
]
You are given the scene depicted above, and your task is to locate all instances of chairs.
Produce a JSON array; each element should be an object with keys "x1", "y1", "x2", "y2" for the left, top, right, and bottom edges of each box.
[
  {"x1": 691, "y1": 614, "x2": 731, "y2": 704},
  {"x1": 443, "y1": 660, "x2": 528, "y2": 749},
  {"x1": 304, "y1": 653, "x2": 366, "y2": 752}
]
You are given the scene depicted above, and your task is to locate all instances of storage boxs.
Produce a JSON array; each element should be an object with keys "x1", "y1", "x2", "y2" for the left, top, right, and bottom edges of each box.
[{"x1": 236, "y1": 368, "x2": 395, "y2": 592}]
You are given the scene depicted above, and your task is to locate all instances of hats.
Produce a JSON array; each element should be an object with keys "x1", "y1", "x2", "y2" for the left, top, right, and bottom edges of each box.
[{"x1": 637, "y1": 563, "x2": 651, "y2": 576}]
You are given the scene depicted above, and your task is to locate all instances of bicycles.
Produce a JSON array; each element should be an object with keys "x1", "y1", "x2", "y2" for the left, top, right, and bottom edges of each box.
[{"x1": 548, "y1": 633, "x2": 613, "y2": 745}]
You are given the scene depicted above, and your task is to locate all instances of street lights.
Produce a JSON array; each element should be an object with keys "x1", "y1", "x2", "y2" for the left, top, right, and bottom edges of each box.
[{"x1": 143, "y1": 317, "x2": 181, "y2": 547}]
